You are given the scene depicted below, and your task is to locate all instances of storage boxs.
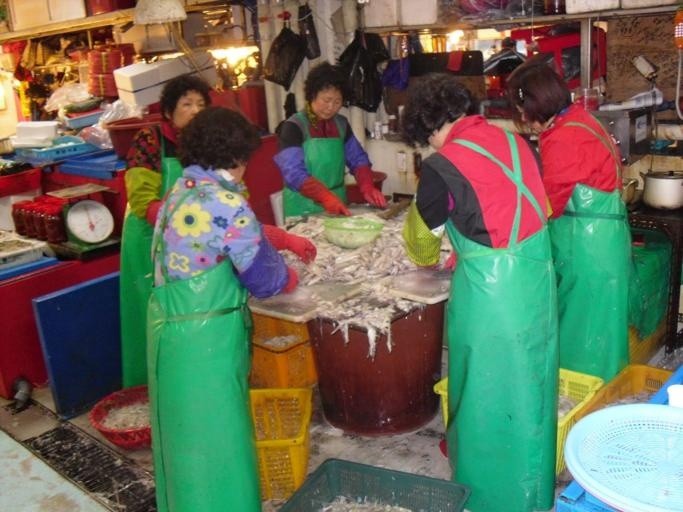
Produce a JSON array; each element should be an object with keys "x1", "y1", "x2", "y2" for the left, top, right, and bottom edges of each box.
[
  {"x1": 251, "y1": 312, "x2": 317, "y2": 393},
  {"x1": 113, "y1": 51, "x2": 216, "y2": 108}
]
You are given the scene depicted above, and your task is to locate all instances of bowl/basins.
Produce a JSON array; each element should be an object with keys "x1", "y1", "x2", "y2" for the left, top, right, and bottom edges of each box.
[
  {"x1": 345, "y1": 171, "x2": 387, "y2": 203},
  {"x1": 322, "y1": 219, "x2": 384, "y2": 248},
  {"x1": 102, "y1": 113, "x2": 162, "y2": 158}
]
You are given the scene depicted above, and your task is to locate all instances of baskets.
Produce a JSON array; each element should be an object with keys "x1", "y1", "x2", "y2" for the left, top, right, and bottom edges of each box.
[
  {"x1": 431, "y1": 363, "x2": 604, "y2": 476},
  {"x1": 240, "y1": 311, "x2": 318, "y2": 393},
  {"x1": 248, "y1": 385, "x2": 313, "y2": 499},
  {"x1": 573, "y1": 364, "x2": 673, "y2": 422},
  {"x1": 564, "y1": 404, "x2": 682, "y2": 510},
  {"x1": 12, "y1": 134, "x2": 86, "y2": 159},
  {"x1": 88, "y1": 382, "x2": 151, "y2": 450},
  {"x1": 276, "y1": 453, "x2": 470, "y2": 510}
]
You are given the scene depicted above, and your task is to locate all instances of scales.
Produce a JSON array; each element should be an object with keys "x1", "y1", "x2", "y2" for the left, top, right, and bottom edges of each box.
[{"x1": 46, "y1": 184, "x2": 121, "y2": 259}]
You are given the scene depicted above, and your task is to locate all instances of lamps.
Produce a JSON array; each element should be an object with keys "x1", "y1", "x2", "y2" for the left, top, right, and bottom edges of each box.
[{"x1": 133, "y1": 0, "x2": 188, "y2": 25}]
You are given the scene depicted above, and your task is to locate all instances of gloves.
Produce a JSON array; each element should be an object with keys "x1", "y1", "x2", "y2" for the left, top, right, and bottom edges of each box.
[
  {"x1": 285, "y1": 268, "x2": 301, "y2": 292},
  {"x1": 445, "y1": 251, "x2": 456, "y2": 272},
  {"x1": 263, "y1": 223, "x2": 317, "y2": 264},
  {"x1": 417, "y1": 262, "x2": 441, "y2": 272},
  {"x1": 354, "y1": 166, "x2": 389, "y2": 208},
  {"x1": 301, "y1": 175, "x2": 351, "y2": 218}
]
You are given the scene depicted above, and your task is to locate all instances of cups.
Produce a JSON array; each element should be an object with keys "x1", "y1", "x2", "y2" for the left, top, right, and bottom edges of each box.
[{"x1": 667, "y1": 385, "x2": 683, "y2": 410}]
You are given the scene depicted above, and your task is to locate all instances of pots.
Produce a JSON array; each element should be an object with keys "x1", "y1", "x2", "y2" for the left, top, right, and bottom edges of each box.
[{"x1": 640, "y1": 169, "x2": 683, "y2": 211}]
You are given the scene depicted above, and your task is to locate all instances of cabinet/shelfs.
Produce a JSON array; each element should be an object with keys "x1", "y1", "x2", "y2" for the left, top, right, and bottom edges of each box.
[{"x1": 6, "y1": 0, "x2": 86, "y2": 32}]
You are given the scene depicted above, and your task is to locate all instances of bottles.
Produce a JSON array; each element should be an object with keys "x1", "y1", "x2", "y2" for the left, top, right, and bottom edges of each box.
[
  {"x1": 13, "y1": 192, "x2": 106, "y2": 242},
  {"x1": 675, "y1": 7, "x2": 683, "y2": 48},
  {"x1": 544, "y1": 0, "x2": 565, "y2": 14},
  {"x1": 373, "y1": 121, "x2": 384, "y2": 140},
  {"x1": 296, "y1": 5, "x2": 321, "y2": 61},
  {"x1": 576, "y1": 89, "x2": 599, "y2": 112}
]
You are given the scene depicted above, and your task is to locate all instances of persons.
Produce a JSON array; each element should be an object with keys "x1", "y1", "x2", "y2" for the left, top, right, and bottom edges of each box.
[
  {"x1": 143, "y1": 102, "x2": 319, "y2": 512},
  {"x1": 272, "y1": 60, "x2": 387, "y2": 225},
  {"x1": 117, "y1": 75, "x2": 250, "y2": 392},
  {"x1": 396, "y1": 71, "x2": 559, "y2": 512},
  {"x1": 502, "y1": 58, "x2": 632, "y2": 385}
]
